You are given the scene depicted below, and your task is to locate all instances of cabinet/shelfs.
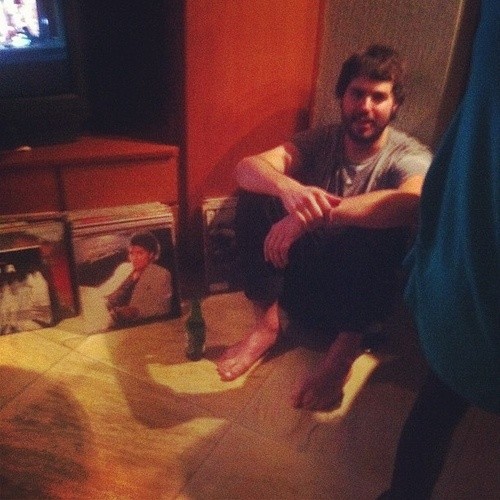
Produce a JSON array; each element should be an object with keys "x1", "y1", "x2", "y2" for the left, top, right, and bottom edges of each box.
[
  {"x1": 164, "y1": 0, "x2": 324, "y2": 278},
  {"x1": 0, "y1": 134, "x2": 178, "y2": 316}
]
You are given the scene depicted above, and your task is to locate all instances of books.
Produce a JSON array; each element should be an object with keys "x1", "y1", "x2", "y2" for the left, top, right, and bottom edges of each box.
[
  {"x1": 202, "y1": 195, "x2": 239, "y2": 293},
  {"x1": 67, "y1": 201, "x2": 182, "y2": 336},
  {"x1": 0, "y1": 212, "x2": 76, "y2": 336}
]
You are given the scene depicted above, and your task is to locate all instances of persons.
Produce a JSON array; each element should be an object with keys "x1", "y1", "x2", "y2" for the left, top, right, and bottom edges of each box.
[
  {"x1": 219, "y1": 45, "x2": 435, "y2": 410},
  {"x1": 1, "y1": 258, "x2": 26, "y2": 334},
  {"x1": 22, "y1": 263, "x2": 54, "y2": 333},
  {"x1": 375, "y1": 0, "x2": 500, "y2": 500},
  {"x1": 79, "y1": 232, "x2": 174, "y2": 332}
]
